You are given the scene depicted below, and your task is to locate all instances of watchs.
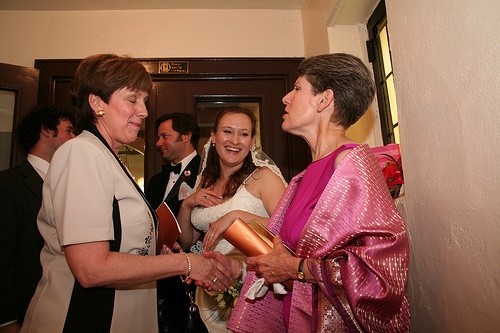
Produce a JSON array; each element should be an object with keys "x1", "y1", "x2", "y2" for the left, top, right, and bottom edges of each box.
[{"x1": 297, "y1": 257, "x2": 307, "y2": 283}]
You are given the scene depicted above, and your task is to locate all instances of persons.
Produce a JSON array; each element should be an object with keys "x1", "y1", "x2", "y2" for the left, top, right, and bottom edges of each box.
[
  {"x1": 177, "y1": 105, "x2": 288, "y2": 333},
  {"x1": 0, "y1": 104, "x2": 76, "y2": 333},
  {"x1": 19, "y1": 53, "x2": 233, "y2": 333},
  {"x1": 184, "y1": 52, "x2": 411, "y2": 333},
  {"x1": 143, "y1": 110, "x2": 202, "y2": 333}
]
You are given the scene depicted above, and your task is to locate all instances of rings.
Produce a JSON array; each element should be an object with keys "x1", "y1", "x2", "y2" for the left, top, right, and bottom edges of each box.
[
  {"x1": 213, "y1": 276, "x2": 218, "y2": 282},
  {"x1": 210, "y1": 234, "x2": 214, "y2": 237},
  {"x1": 203, "y1": 193, "x2": 210, "y2": 199}
]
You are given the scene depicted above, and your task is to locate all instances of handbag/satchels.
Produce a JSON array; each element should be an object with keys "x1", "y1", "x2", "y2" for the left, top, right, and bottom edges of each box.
[{"x1": 160, "y1": 281, "x2": 209, "y2": 333}]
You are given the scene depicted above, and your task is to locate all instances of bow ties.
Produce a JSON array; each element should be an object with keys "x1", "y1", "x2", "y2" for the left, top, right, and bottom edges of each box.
[{"x1": 161, "y1": 162, "x2": 182, "y2": 174}]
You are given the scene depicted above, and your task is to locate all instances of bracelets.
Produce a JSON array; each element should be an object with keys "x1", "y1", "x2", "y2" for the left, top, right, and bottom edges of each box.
[{"x1": 179, "y1": 251, "x2": 192, "y2": 282}]
